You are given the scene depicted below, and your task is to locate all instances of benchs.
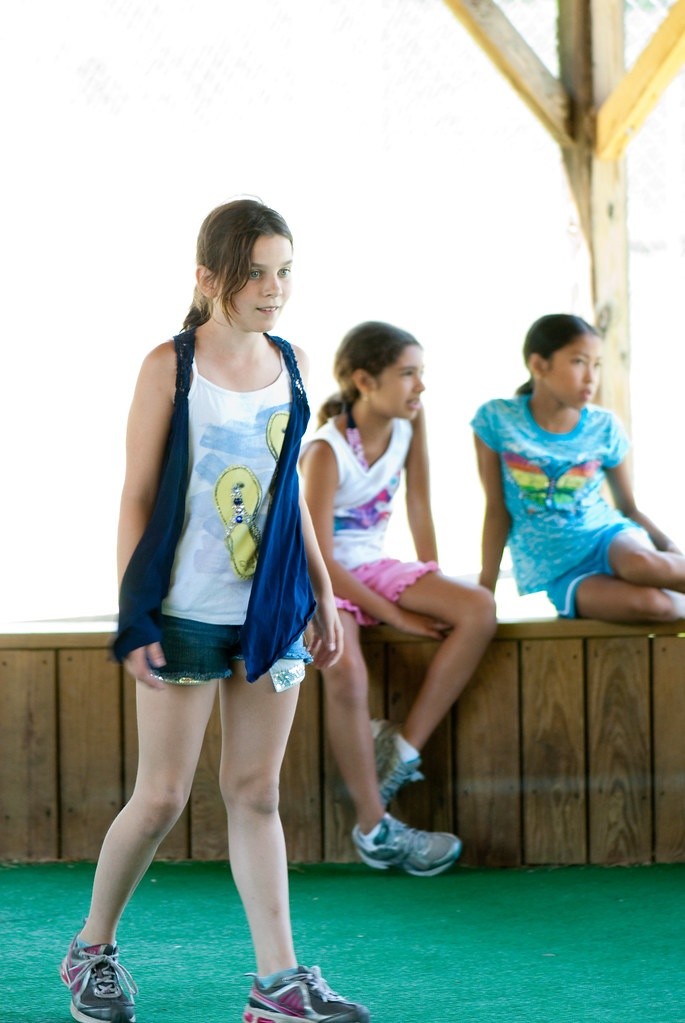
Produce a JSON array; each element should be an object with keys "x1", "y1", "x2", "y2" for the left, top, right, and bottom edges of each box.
[{"x1": 0, "y1": 607, "x2": 685, "y2": 864}]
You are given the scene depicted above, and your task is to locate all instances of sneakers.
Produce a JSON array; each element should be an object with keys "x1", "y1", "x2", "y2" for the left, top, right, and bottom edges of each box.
[
  {"x1": 368, "y1": 717, "x2": 424, "y2": 810},
  {"x1": 351, "y1": 812, "x2": 462, "y2": 877},
  {"x1": 243, "y1": 966, "x2": 371, "y2": 1023},
  {"x1": 59, "y1": 926, "x2": 137, "y2": 1023}
]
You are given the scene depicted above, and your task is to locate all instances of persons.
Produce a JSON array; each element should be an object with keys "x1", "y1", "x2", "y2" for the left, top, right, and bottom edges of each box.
[
  {"x1": 298, "y1": 320, "x2": 496, "y2": 876},
  {"x1": 58, "y1": 198, "x2": 373, "y2": 1023},
  {"x1": 469, "y1": 313, "x2": 684, "y2": 618}
]
